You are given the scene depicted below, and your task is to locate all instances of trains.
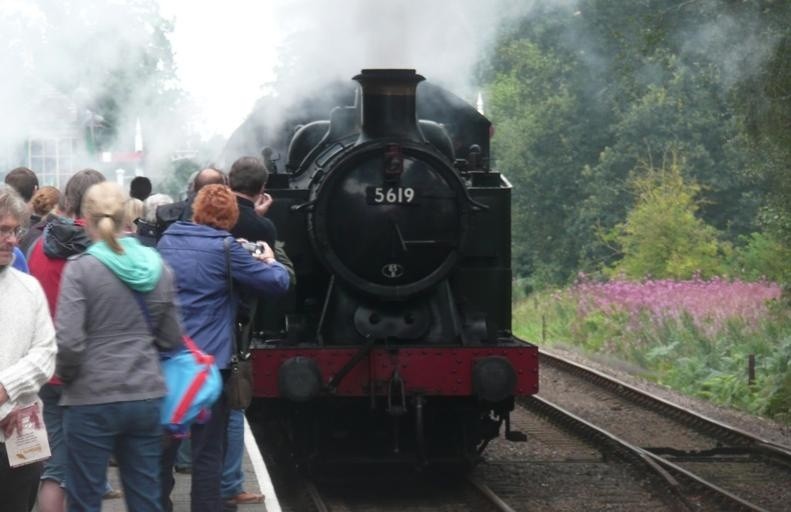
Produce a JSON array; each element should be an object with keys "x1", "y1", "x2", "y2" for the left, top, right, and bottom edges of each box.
[{"x1": 163, "y1": 59, "x2": 541, "y2": 494}]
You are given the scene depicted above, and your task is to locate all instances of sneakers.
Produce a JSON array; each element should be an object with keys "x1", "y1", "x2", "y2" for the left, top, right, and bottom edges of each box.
[
  {"x1": 101, "y1": 489, "x2": 124, "y2": 500},
  {"x1": 189, "y1": 492, "x2": 265, "y2": 512}
]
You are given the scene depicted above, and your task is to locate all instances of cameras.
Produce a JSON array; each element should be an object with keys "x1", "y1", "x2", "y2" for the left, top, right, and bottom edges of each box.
[{"x1": 242, "y1": 241, "x2": 262, "y2": 253}]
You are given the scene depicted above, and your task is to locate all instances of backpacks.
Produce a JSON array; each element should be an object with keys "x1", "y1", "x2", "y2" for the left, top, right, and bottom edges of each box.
[{"x1": 156, "y1": 335, "x2": 223, "y2": 433}]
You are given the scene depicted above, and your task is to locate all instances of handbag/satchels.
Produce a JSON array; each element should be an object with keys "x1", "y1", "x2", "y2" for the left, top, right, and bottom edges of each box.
[{"x1": 227, "y1": 352, "x2": 257, "y2": 411}]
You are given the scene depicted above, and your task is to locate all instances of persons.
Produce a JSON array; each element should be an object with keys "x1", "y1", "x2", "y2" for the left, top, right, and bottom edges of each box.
[
  {"x1": 53, "y1": 181, "x2": 183, "y2": 511},
  {"x1": 1, "y1": 168, "x2": 105, "y2": 511},
  {"x1": 129, "y1": 156, "x2": 297, "y2": 512}
]
